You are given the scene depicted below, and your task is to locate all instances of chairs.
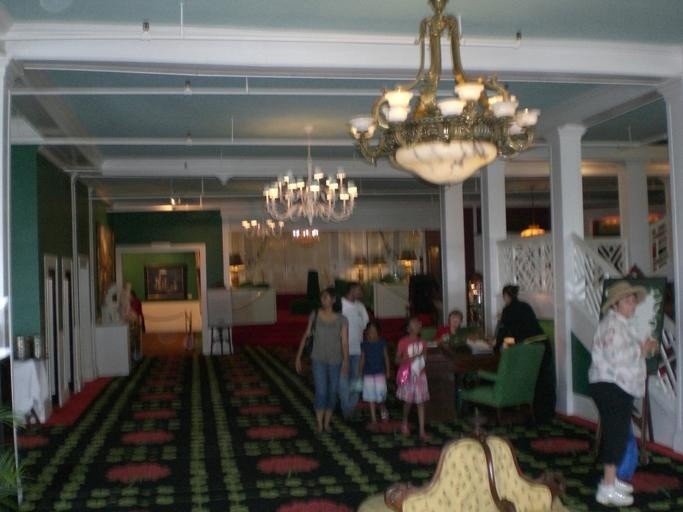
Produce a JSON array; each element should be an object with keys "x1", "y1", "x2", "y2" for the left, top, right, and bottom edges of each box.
[
  {"x1": 384, "y1": 434, "x2": 567, "y2": 512},
  {"x1": 457, "y1": 344, "x2": 545, "y2": 430}
]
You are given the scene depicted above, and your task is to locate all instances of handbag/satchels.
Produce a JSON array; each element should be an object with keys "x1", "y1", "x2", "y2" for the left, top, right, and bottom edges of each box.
[{"x1": 304, "y1": 308, "x2": 319, "y2": 362}]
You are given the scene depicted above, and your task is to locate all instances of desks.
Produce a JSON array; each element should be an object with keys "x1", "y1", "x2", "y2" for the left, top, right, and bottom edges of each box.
[{"x1": 423, "y1": 337, "x2": 502, "y2": 371}]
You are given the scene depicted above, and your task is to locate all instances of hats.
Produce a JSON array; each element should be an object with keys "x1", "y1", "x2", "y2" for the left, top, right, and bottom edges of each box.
[{"x1": 602, "y1": 281, "x2": 646, "y2": 314}]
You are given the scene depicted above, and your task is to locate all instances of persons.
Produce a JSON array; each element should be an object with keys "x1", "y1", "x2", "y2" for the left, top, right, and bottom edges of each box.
[
  {"x1": 338, "y1": 282, "x2": 369, "y2": 423},
  {"x1": 433, "y1": 310, "x2": 464, "y2": 345},
  {"x1": 494, "y1": 285, "x2": 558, "y2": 421},
  {"x1": 394, "y1": 314, "x2": 433, "y2": 442},
  {"x1": 359, "y1": 320, "x2": 392, "y2": 425},
  {"x1": 586, "y1": 280, "x2": 660, "y2": 507},
  {"x1": 294, "y1": 287, "x2": 350, "y2": 433}
]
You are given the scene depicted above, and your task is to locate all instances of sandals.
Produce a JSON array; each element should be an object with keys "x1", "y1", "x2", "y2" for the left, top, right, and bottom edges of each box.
[{"x1": 380, "y1": 409, "x2": 389, "y2": 422}]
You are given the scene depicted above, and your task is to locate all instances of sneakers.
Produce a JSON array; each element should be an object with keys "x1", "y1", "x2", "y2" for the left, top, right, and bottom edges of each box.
[
  {"x1": 596, "y1": 484, "x2": 633, "y2": 507},
  {"x1": 601, "y1": 476, "x2": 633, "y2": 493},
  {"x1": 400, "y1": 424, "x2": 411, "y2": 437},
  {"x1": 418, "y1": 433, "x2": 431, "y2": 442}
]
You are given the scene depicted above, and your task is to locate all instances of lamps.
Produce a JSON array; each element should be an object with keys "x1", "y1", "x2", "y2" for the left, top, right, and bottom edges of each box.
[
  {"x1": 373, "y1": 254, "x2": 388, "y2": 280},
  {"x1": 348, "y1": 0, "x2": 543, "y2": 189},
  {"x1": 229, "y1": 252, "x2": 244, "y2": 286},
  {"x1": 240, "y1": 218, "x2": 284, "y2": 241},
  {"x1": 290, "y1": 223, "x2": 320, "y2": 245},
  {"x1": 262, "y1": 127, "x2": 358, "y2": 227},
  {"x1": 398, "y1": 248, "x2": 417, "y2": 266},
  {"x1": 353, "y1": 254, "x2": 368, "y2": 283},
  {"x1": 520, "y1": 185, "x2": 546, "y2": 237}
]
